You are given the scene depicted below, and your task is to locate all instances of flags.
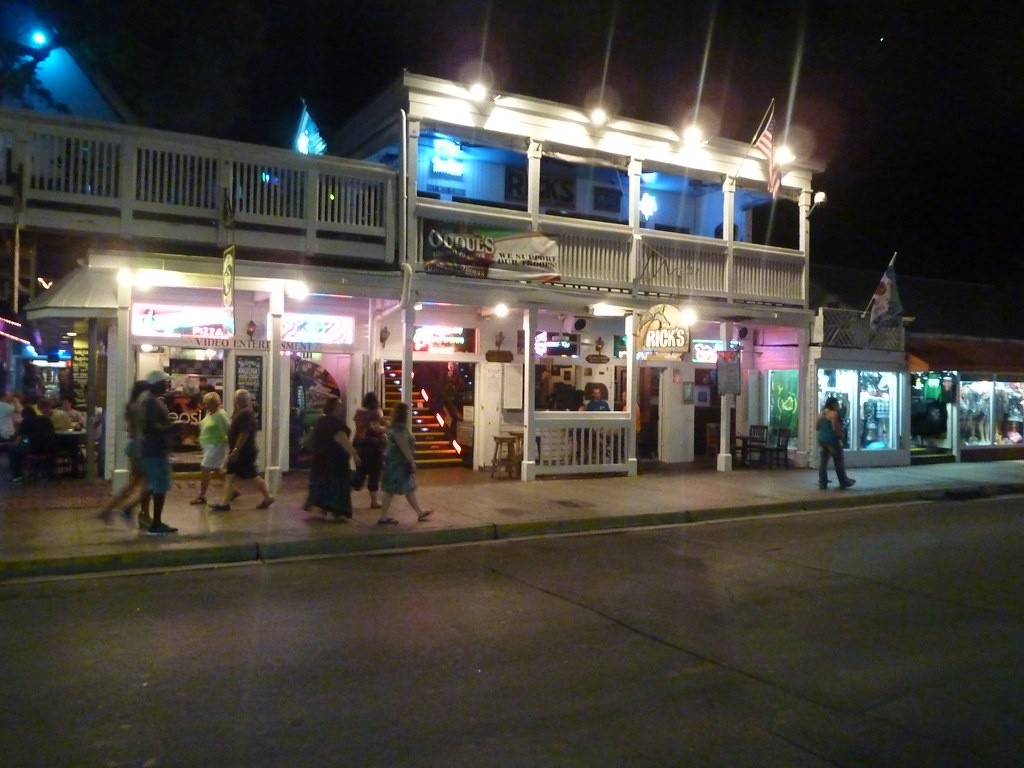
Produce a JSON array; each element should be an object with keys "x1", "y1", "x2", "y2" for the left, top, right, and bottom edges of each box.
[
  {"x1": 755, "y1": 112, "x2": 784, "y2": 199},
  {"x1": 870, "y1": 264, "x2": 904, "y2": 329}
]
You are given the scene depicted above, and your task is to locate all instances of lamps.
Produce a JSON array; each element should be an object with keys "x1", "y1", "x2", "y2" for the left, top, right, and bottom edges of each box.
[
  {"x1": 595, "y1": 336, "x2": 604, "y2": 355},
  {"x1": 495, "y1": 331, "x2": 505, "y2": 347},
  {"x1": 247, "y1": 320, "x2": 257, "y2": 341},
  {"x1": 380, "y1": 326, "x2": 390, "y2": 348},
  {"x1": 806, "y1": 191, "x2": 826, "y2": 219}
]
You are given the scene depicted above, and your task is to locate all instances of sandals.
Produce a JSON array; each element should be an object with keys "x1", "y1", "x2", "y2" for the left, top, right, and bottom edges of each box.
[{"x1": 191, "y1": 496, "x2": 206, "y2": 503}]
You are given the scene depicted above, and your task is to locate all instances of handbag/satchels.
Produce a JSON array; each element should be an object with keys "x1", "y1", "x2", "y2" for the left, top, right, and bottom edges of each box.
[{"x1": 363, "y1": 430, "x2": 388, "y2": 450}]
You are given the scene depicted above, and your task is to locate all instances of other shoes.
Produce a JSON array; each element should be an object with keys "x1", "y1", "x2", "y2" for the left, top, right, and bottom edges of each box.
[
  {"x1": 137, "y1": 514, "x2": 150, "y2": 528},
  {"x1": 255, "y1": 497, "x2": 276, "y2": 510},
  {"x1": 210, "y1": 502, "x2": 233, "y2": 511},
  {"x1": 147, "y1": 523, "x2": 177, "y2": 535}
]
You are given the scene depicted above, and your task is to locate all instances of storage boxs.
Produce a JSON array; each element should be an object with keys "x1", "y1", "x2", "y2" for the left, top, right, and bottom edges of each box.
[{"x1": 456, "y1": 406, "x2": 474, "y2": 446}]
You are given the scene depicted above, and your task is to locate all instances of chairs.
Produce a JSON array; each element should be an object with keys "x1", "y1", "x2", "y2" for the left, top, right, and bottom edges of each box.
[
  {"x1": 748, "y1": 425, "x2": 768, "y2": 468},
  {"x1": 759, "y1": 428, "x2": 791, "y2": 469},
  {"x1": 705, "y1": 423, "x2": 719, "y2": 466}
]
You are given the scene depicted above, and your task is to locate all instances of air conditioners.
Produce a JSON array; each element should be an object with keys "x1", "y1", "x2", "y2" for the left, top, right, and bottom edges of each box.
[
  {"x1": 563, "y1": 312, "x2": 594, "y2": 335},
  {"x1": 733, "y1": 324, "x2": 755, "y2": 341}
]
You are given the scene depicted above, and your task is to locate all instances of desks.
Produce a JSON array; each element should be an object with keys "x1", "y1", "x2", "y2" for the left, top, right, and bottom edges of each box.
[
  {"x1": 507, "y1": 431, "x2": 542, "y2": 479},
  {"x1": 736, "y1": 434, "x2": 760, "y2": 469}
]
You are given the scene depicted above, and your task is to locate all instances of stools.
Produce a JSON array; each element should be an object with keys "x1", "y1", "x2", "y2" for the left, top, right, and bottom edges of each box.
[{"x1": 490, "y1": 435, "x2": 517, "y2": 479}]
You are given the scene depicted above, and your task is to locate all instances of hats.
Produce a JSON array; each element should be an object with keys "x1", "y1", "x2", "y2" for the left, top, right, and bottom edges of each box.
[{"x1": 148, "y1": 369, "x2": 168, "y2": 384}]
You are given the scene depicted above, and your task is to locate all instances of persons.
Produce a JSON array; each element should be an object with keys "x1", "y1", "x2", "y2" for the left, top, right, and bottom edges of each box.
[
  {"x1": 97, "y1": 370, "x2": 275, "y2": 535},
  {"x1": 302, "y1": 392, "x2": 434, "y2": 523},
  {"x1": 535, "y1": 368, "x2": 641, "y2": 434},
  {"x1": 0, "y1": 365, "x2": 87, "y2": 481},
  {"x1": 815, "y1": 397, "x2": 854, "y2": 488}
]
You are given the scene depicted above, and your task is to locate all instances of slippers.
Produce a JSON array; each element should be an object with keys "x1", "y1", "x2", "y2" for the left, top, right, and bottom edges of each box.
[
  {"x1": 419, "y1": 508, "x2": 431, "y2": 520},
  {"x1": 378, "y1": 518, "x2": 398, "y2": 526}
]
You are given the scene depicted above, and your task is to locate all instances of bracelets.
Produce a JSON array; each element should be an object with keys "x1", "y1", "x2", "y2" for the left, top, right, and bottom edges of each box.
[{"x1": 233, "y1": 448, "x2": 240, "y2": 456}]
